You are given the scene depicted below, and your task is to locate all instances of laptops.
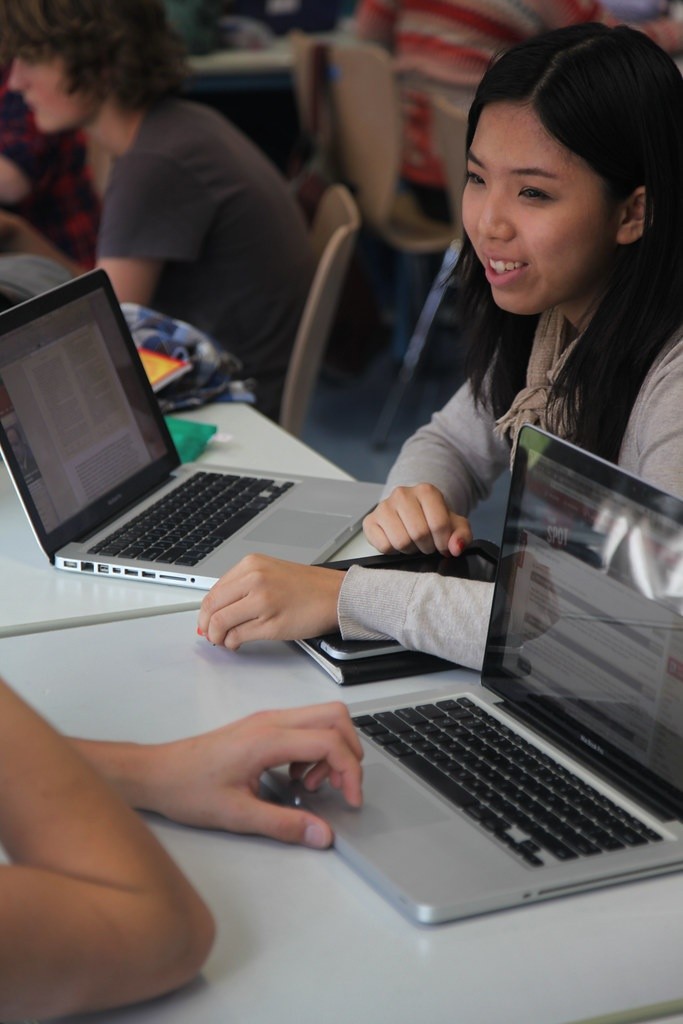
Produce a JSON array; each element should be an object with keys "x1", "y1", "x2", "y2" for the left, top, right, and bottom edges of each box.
[
  {"x1": 258, "y1": 423, "x2": 683, "y2": 925},
  {"x1": 0, "y1": 268, "x2": 387, "y2": 591}
]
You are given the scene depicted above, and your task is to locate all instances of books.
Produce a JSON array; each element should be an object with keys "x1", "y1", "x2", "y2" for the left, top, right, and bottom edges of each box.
[{"x1": 293, "y1": 545, "x2": 499, "y2": 685}]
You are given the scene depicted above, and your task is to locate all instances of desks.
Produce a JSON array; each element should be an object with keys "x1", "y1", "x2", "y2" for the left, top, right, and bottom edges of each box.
[
  {"x1": 186, "y1": 43, "x2": 296, "y2": 92},
  {"x1": 1, "y1": 610, "x2": 683, "y2": 1024},
  {"x1": 0, "y1": 402, "x2": 408, "y2": 634}
]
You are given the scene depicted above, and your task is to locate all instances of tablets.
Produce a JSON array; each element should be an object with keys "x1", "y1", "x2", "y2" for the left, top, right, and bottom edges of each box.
[{"x1": 311, "y1": 547, "x2": 497, "y2": 660}]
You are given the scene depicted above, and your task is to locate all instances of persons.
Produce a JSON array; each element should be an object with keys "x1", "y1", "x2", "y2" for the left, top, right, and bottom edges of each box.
[
  {"x1": 350, "y1": 0, "x2": 683, "y2": 227},
  {"x1": 0, "y1": 675, "x2": 365, "y2": 1024},
  {"x1": 197, "y1": 21, "x2": 683, "y2": 673},
  {"x1": 0, "y1": 0, "x2": 317, "y2": 421}
]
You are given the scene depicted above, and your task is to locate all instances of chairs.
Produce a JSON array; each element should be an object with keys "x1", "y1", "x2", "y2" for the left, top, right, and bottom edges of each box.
[
  {"x1": 282, "y1": 184, "x2": 360, "y2": 435},
  {"x1": 426, "y1": 88, "x2": 469, "y2": 234},
  {"x1": 291, "y1": 34, "x2": 462, "y2": 376}
]
